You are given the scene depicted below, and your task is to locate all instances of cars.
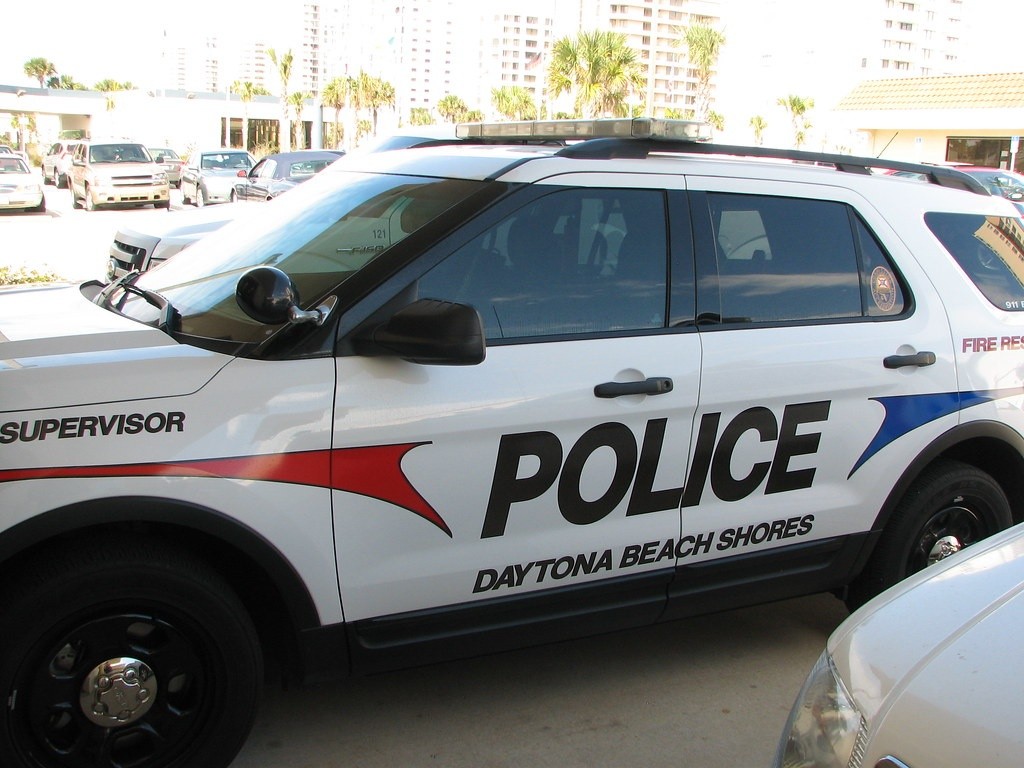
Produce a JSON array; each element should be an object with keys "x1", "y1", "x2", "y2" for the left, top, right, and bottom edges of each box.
[
  {"x1": 0, "y1": 145, "x2": 44, "y2": 212},
  {"x1": 230, "y1": 149, "x2": 345, "y2": 204},
  {"x1": 145, "y1": 148, "x2": 183, "y2": 188},
  {"x1": 184, "y1": 149, "x2": 261, "y2": 207}
]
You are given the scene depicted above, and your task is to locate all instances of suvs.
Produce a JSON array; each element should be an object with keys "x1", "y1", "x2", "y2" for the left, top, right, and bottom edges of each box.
[
  {"x1": 67, "y1": 139, "x2": 171, "y2": 211},
  {"x1": 0, "y1": 116, "x2": 1024, "y2": 767},
  {"x1": 41, "y1": 140, "x2": 82, "y2": 189}
]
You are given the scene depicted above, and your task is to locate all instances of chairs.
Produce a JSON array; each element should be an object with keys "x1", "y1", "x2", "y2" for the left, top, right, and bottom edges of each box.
[
  {"x1": 380, "y1": 195, "x2": 513, "y2": 348},
  {"x1": 477, "y1": 214, "x2": 626, "y2": 348}
]
